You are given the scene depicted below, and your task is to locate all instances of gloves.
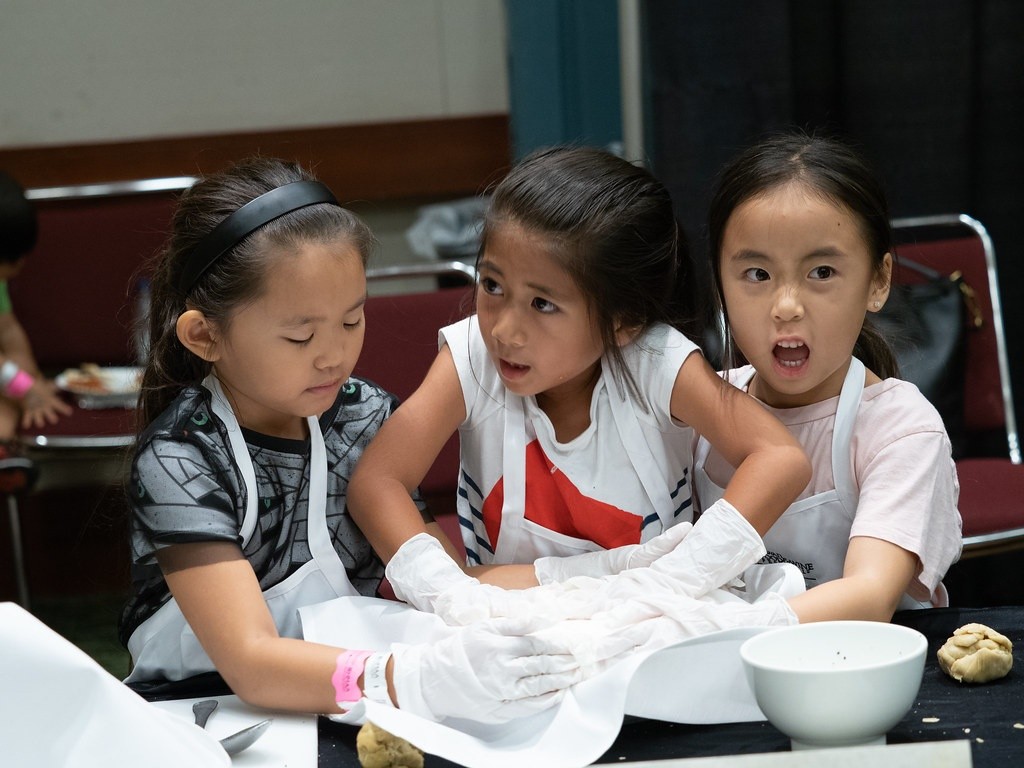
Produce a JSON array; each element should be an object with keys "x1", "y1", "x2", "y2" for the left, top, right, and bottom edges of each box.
[{"x1": 379, "y1": 498, "x2": 804, "y2": 750}]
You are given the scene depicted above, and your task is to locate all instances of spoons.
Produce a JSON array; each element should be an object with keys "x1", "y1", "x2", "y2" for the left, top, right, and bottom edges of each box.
[
  {"x1": 188, "y1": 699, "x2": 218, "y2": 730},
  {"x1": 217, "y1": 717, "x2": 273, "y2": 756}
]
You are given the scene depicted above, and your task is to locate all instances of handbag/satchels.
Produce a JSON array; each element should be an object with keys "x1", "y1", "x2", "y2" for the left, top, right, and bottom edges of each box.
[{"x1": 867, "y1": 253, "x2": 982, "y2": 439}]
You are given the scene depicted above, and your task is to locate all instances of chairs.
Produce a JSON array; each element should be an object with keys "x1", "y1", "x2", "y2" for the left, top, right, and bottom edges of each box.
[{"x1": 2, "y1": 177, "x2": 1024, "y2": 571}]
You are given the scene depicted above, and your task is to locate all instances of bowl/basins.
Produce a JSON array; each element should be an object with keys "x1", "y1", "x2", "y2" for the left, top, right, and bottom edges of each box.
[{"x1": 738, "y1": 621, "x2": 929, "y2": 755}]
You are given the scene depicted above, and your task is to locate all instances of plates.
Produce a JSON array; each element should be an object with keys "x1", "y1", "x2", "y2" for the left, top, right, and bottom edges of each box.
[{"x1": 52, "y1": 366, "x2": 154, "y2": 410}]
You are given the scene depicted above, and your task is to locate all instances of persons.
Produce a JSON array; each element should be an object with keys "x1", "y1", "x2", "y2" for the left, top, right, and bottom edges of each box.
[
  {"x1": 127, "y1": 149, "x2": 570, "y2": 720},
  {"x1": 343, "y1": 134, "x2": 815, "y2": 643},
  {"x1": 1, "y1": 167, "x2": 75, "y2": 444},
  {"x1": 551, "y1": 136, "x2": 963, "y2": 685}
]
